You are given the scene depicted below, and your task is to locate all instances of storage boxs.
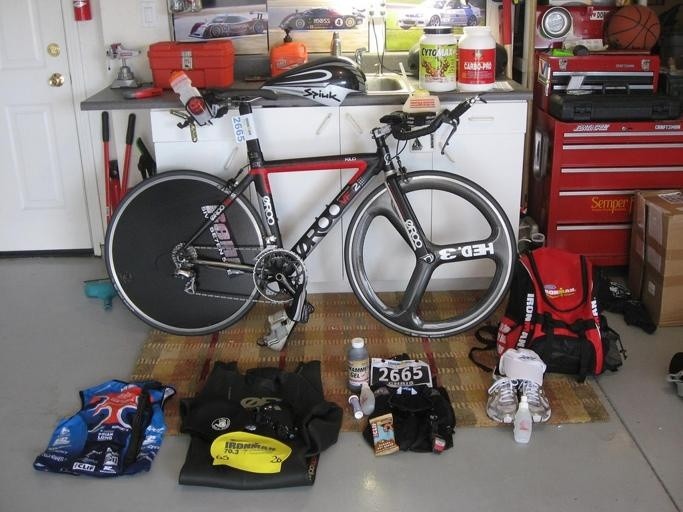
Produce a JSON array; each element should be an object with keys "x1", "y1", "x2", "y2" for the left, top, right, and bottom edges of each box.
[
  {"x1": 147, "y1": 41, "x2": 234, "y2": 89},
  {"x1": 627, "y1": 189, "x2": 683, "y2": 327}
]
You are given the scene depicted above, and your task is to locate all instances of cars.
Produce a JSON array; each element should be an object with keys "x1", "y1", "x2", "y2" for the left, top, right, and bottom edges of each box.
[
  {"x1": 279, "y1": 8, "x2": 364, "y2": 30},
  {"x1": 398, "y1": 0, "x2": 481, "y2": 30},
  {"x1": 188, "y1": 10, "x2": 268, "y2": 38}
]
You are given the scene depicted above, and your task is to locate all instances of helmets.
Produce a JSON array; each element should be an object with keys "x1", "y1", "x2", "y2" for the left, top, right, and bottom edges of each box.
[{"x1": 258, "y1": 53, "x2": 369, "y2": 111}]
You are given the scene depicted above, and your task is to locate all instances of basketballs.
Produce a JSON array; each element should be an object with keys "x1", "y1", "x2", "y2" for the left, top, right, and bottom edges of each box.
[{"x1": 607, "y1": 6, "x2": 661, "y2": 51}]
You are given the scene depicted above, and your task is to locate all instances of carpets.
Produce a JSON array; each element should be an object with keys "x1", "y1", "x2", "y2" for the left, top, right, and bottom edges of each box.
[{"x1": 135, "y1": 289, "x2": 611, "y2": 436}]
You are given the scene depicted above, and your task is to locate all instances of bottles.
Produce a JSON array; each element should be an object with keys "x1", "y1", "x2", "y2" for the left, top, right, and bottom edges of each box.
[
  {"x1": 514, "y1": 395, "x2": 532, "y2": 444},
  {"x1": 458, "y1": 26, "x2": 497, "y2": 92},
  {"x1": 168, "y1": 70, "x2": 212, "y2": 126},
  {"x1": 418, "y1": 25, "x2": 458, "y2": 94},
  {"x1": 347, "y1": 337, "x2": 370, "y2": 393},
  {"x1": 359, "y1": 380, "x2": 375, "y2": 416}
]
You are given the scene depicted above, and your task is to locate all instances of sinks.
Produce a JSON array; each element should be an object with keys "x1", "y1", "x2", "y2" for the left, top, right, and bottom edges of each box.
[{"x1": 364, "y1": 73, "x2": 412, "y2": 96}]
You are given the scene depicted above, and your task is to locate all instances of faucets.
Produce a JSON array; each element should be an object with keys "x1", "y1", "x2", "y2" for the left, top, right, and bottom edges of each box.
[{"x1": 355, "y1": 47, "x2": 368, "y2": 68}]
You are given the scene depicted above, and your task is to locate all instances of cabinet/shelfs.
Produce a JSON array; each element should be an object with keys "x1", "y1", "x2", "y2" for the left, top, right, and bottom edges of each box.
[{"x1": 148, "y1": 99, "x2": 528, "y2": 296}]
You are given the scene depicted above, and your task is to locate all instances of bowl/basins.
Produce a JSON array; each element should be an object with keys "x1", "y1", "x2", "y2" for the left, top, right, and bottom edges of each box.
[{"x1": 541, "y1": 6, "x2": 574, "y2": 39}]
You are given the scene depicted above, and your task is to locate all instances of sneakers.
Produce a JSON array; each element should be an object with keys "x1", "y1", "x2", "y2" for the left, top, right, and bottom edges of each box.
[
  {"x1": 520, "y1": 379, "x2": 552, "y2": 423},
  {"x1": 262, "y1": 307, "x2": 298, "y2": 353},
  {"x1": 485, "y1": 365, "x2": 520, "y2": 423}
]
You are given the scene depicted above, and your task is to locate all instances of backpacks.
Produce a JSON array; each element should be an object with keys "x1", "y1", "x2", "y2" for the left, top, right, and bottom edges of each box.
[
  {"x1": 363, "y1": 383, "x2": 456, "y2": 456},
  {"x1": 468, "y1": 236, "x2": 627, "y2": 386}
]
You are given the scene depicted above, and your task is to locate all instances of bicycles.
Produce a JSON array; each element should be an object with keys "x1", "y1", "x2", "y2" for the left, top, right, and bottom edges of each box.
[{"x1": 104, "y1": 87, "x2": 518, "y2": 341}]
